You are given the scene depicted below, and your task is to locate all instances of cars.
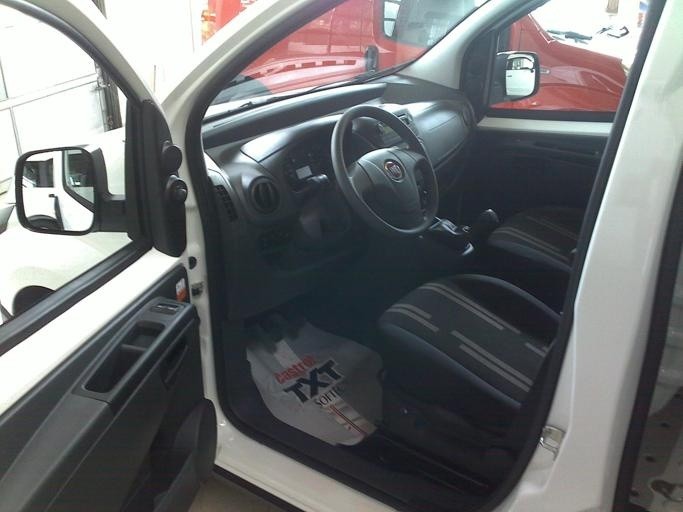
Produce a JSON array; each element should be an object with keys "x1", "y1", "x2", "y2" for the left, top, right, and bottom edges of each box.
[
  {"x1": 201, "y1": 0, "x2": 629, "y2": 113},
  {"x1": 1, "y1": 0, "x2": 682, "y2": 512}
]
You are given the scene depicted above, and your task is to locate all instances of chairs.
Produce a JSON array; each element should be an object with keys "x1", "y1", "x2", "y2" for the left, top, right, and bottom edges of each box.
[
  {"x1": 477, "y1": 206, "x2": 585, "y2": 314},
  {"x1": 371, "y1": 272, "x2": 561, "y2": 497}
]
